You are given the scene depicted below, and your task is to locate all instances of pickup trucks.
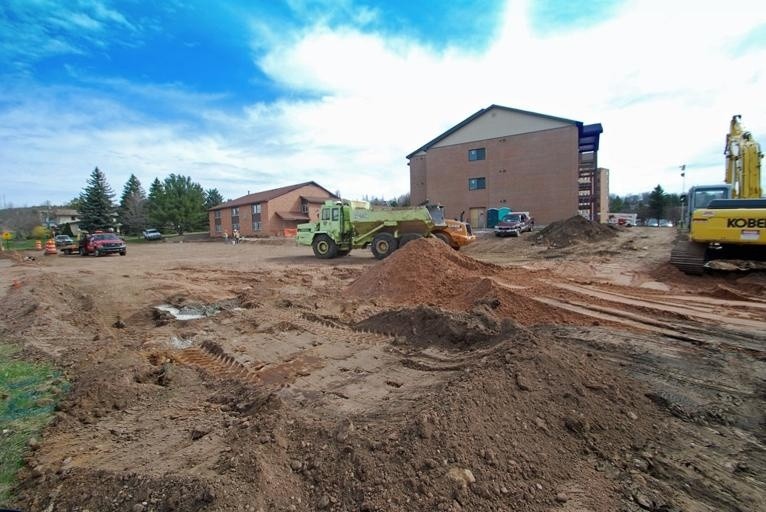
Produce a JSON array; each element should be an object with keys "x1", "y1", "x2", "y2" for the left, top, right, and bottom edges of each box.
[{"x1": 76, "y1": 230, "x2": 130, "y2": 257}]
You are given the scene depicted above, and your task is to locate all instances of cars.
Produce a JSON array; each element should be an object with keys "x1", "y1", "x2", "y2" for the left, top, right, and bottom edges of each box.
[
  {"x1": 142, "y1": 228, "x2": 162, "y2": 241},
  {"x1": 647, "y1": 218, "x2": 675, "y2": 228},
  {"x1": 50, "y1": 234, "x2": 75, "y2": 246},
  {"x1": 494, "y1": 211, "x2": 536, "y2": 238}
]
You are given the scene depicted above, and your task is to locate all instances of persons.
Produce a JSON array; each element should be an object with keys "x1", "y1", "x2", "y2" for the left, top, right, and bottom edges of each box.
[
  {"x1": 224, "y1": 228, "x2": 228, "y2": 244},
  {"x1": 232, "y1": 228, "x2": 240, "y2": 244},
  {"x1": 177, "y1": 224, "x2": 185, "y2": 244},
  {"x1": 82, "y1": 234, "x2": 91, "y2": 256}
]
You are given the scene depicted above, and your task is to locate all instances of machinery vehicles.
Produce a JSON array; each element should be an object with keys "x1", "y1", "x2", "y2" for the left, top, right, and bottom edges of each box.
[
  {"x1": 294, "y1": 197, "x2": 447, "y2": 261},
  {"x1": 665, "y1": 114, "x2": 766, "y2": 279},
  {"x1": 437, "y1": 209, "x2": 479, "y2": 251}
]
